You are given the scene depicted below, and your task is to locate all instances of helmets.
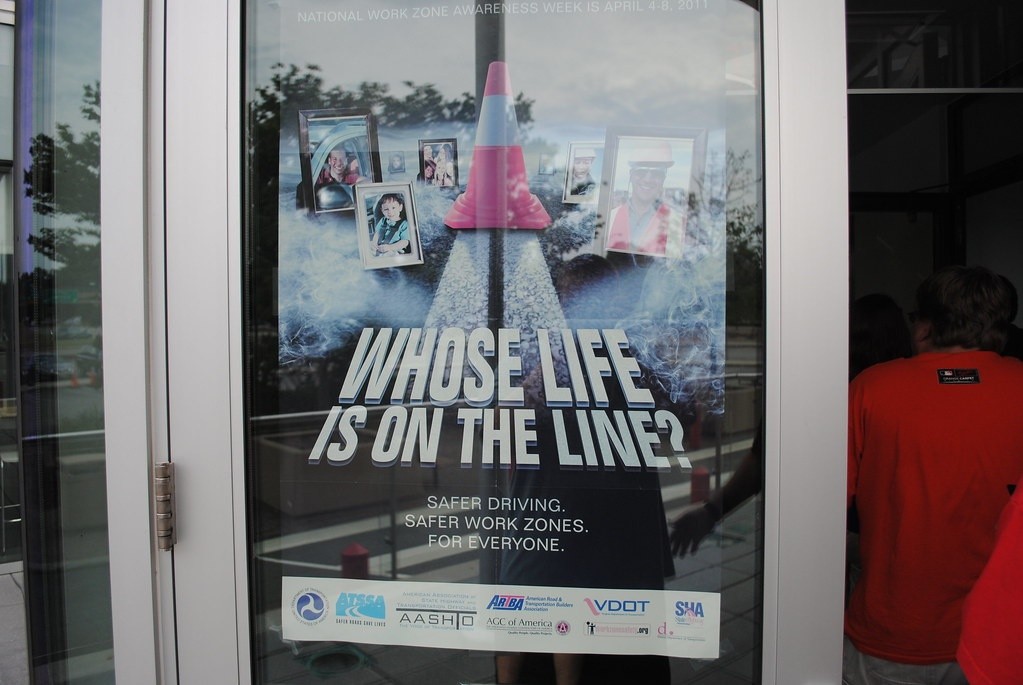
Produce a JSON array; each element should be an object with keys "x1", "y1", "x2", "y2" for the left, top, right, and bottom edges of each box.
[
  {"x1": 574, "y1": 148, "x2": 596, "y2": 161},
  {"x1": 626, "y1": 139, "x2": 675, "y2": 168}
]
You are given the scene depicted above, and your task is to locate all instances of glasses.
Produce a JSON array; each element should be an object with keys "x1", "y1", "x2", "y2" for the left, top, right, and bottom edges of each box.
[
  {"x1": 906, "y1": 311, "x2": 922, "y2": 323},
  {"x1": 631, "y1": 166, "x2": 666, "y2": 178}
]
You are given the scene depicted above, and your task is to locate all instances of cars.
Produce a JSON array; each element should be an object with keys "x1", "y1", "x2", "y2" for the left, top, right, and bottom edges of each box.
[{"x1": 22, "y1": 350, "x2": 76, "y2": 386}]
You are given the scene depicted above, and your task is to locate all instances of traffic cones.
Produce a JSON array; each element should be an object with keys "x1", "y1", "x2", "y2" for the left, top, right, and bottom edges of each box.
[{"x1": 446, "y1": 61, "x2": 554, "y2": 233}]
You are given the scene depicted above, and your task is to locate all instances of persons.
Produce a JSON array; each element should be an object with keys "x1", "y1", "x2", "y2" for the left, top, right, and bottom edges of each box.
[
  {"x1": 955, "y1": 468, "x2": 1023, "y2": 685},
  {"x1": 369, "y1": 194, "x2": 410, "y2": 256},
  {"x1": 423, "y1": 145, "x2": 434, "y2": 161},
  {"x1": 846, "y1": 260, "x2": 1023, "y2": 685},
  {"x1": 434, "y1": 144, "x2": 453, "y2": 186},
  {"x1": 424, "y1": 160, "x2": 436, "y2": 185},
  {"x1": 667, "y1": 419, "x2": 762, "y2": 557},
  {"x1": 848, "y1": 292, "x2": 915, "y2": 553},
  {"x1": 316, "y1": 146, "x2": 368, "y2": 184},
  {"x1": 477, "y1": 252, "x2": 676, "y2": 685},
  {"x1": 570, "y1": 146, "x2": 596, "y2": 197},
  {"x1": 605, "y1": 140, "x2": 684, "y2": 257},
  {"x1": 392, "y1": 155, "x2": 403, "y2": 170}
]
